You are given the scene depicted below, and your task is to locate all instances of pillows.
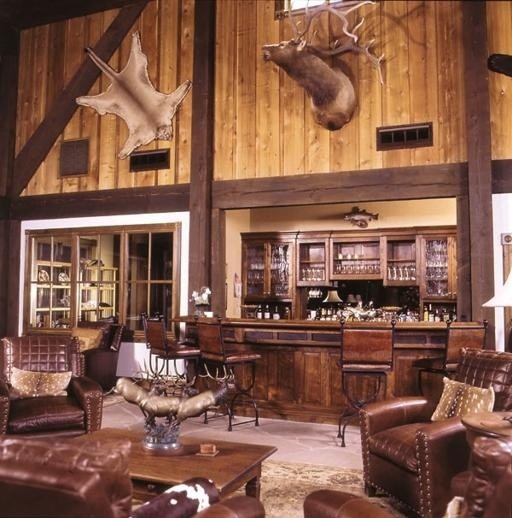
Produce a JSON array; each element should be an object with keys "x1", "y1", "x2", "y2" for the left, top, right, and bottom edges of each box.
[
  {"x1": 431, "y1": 377, "x2": 495, "y2": 422},
  {"x1": 133, "y1": 477, "x2": 220, "y2": 518},
  {"x1": 9, "y1": 367, "x2": 72, "y2": 401}
]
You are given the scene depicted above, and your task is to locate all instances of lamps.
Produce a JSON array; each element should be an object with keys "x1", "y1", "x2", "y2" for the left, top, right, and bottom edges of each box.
[{"x1": 323, "y1": 290, "x2": 343, "y2": 319}]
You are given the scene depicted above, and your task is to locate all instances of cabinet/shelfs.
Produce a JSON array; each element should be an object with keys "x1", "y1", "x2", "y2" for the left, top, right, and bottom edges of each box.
[
  {"x1": 36, "y1": 260, "x2": 118, "y2": 328},
  {"x1": 383, "y1": 235, "x2": 420, "y2": 286},
  {"x1": 329, "y1": 237, "x2": 384, "y2": 281},
  {"x1": 423, "y1": 302, "x2": 457, "y2": 322},
  {"x1": 420, "y1": 234, "x2": 458, "y2": 300},
  {"x1": 241, "y1": 239, "x2": 296, "y2": 299},
  {"x1": 297, "y1": 238, "x2": 333, "y2": 287},
  {"x1": 244, "y1": 301, "x2": 294, "y2": 320}
]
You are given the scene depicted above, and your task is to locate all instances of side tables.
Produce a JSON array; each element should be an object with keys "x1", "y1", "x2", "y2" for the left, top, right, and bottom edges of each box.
[{"x1": 461, "y1": 411, "x2": 512, "y2": 450}]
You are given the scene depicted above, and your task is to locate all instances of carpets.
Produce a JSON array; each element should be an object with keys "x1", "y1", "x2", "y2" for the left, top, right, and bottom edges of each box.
[{"x1": 225, "y1": 459, "x2": 404, "y2": 518}]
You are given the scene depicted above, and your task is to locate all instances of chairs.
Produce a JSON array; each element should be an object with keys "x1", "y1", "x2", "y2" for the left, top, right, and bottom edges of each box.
[
  {"x1": 337, "y1": 318, "x2": 396, "y2": 447},
  {"x1": 303, "y1": 437, "x2": 512, "y2": 518},
  {"x1": 193, "y1": 314, "x2": 261, "y2": 431},
  {"x1": 0, "y1": 334, "x2": 103, "y2": 436},
  {"x1": 359, "y1": 347, "x2": 512, "y2": 518},
  {"x1": 412, "y1": 319, "x2": 489, "y2": 395},
  {"x1": 145, "y1": 315, "x2": 201, "y2": 391}
]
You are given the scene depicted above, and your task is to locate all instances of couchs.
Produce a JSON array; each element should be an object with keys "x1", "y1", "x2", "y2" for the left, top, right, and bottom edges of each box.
[{"x1": 0, "y1": 434, "x2": 265, "y2": 518}]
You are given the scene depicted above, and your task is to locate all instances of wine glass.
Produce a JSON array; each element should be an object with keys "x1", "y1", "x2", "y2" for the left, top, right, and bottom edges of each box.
[{"x1": 425, "y1": 239, "x2": 448, "y2": 295}]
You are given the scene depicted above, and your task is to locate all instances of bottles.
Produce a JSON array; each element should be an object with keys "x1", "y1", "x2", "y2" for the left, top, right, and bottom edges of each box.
[
  {"x1": 306, "y1": 288, "x2": 456, "y2": 322},
  {"x1": 248, "y1": 243, "x2": 289, "y2": 320},
  {"x1": 302, "y1": 266, "x2": 325, "y2": 281},
  {"x1": 335, "y1": 254, "x2": 380, "y2": 273},
  {"x1": 388, "y1": 266, "x2": 416, "y2": 281}
]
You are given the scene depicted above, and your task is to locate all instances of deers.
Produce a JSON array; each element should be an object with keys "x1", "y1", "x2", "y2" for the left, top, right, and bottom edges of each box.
[
  {"x1": 261, "y1": 0, "x2": 385, "y2": 130},
  {"x1": 168, "y1": 363, "x2": 237, "y2": 431},
  {"x1": 102, "y1": 357, "x2": 169, "y2": 405},
  {"x1": 139, "y1": 371, "x2": 200, "y2": 426}
]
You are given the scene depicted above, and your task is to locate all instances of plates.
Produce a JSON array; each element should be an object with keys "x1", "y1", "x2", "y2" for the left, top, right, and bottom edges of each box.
[{"x1": 38, "y1": 270, "x2": 69, "y2": 286}]
